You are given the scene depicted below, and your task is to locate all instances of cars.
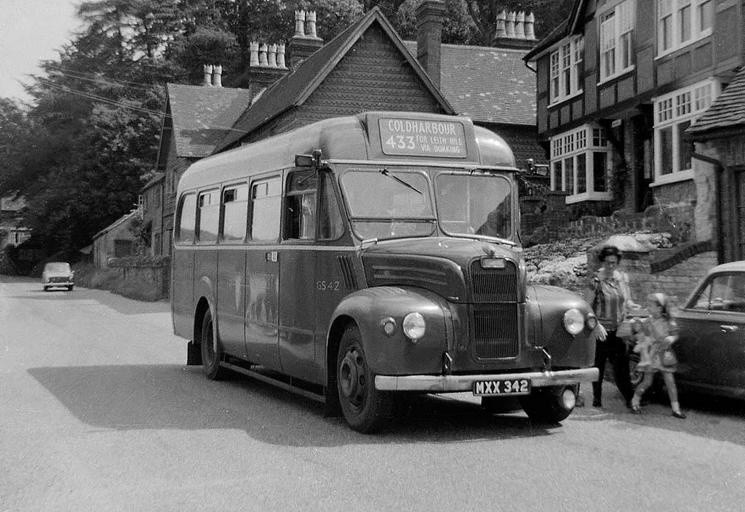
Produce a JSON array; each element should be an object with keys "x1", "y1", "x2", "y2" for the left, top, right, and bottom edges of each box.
[
  {"x1": 622, "y1": 259, "x2": 744, "y2": 424},
  {"x1": 41, "y1": 262, "x2": 74, "y2": 291}
]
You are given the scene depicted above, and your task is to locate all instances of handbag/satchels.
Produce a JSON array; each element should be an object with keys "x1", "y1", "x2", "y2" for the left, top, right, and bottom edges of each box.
[
  {"x1": 615, "y1": 318, "x2": 641, "y2": 338},
  {"x1": 662, "y1": 350, "x2": 678, "y2": 366}
]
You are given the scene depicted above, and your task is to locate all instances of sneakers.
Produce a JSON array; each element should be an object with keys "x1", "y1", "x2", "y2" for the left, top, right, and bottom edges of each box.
[{"x1": 593, "y1": 401, "x2": 687, "y2": 418}]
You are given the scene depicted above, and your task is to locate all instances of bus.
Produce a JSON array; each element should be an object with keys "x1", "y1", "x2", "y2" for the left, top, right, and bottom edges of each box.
[{"x1": 168, "y1": 112, "x2": 600, "y2": 433}]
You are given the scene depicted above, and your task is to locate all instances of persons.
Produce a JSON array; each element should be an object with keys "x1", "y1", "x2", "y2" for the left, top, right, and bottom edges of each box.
[
  {"x1": 626, "y1": 292, "x2": 687, "y2": 419},
  {"x1": 583, "y1": 243, "x2": 642, "y2": 408}
]
